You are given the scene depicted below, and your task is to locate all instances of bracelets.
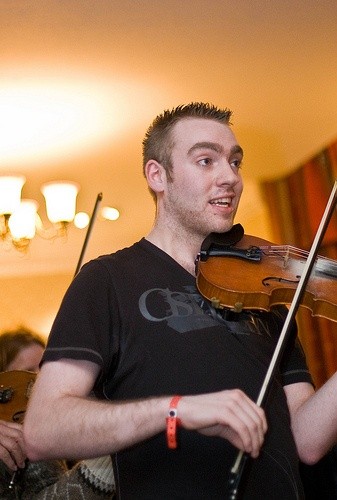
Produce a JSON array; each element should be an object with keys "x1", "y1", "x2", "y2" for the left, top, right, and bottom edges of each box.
[{"x1": 165, "y1": 394, "x2": 182, "y2": 448}]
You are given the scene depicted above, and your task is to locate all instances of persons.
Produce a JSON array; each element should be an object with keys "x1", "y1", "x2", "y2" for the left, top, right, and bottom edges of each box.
[
  {"x1": 0, "y1": 327, "x2": 117, "y2": 500},
  {"x1": 22, "y1": 102, "x2": 337, "y2": 500}
]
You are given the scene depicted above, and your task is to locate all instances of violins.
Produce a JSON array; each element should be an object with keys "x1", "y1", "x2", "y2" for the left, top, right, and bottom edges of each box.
[
  {"x1": 0, "y1": 370, "x2": 38, "y2": 424},
  {"x1": 193, "y1": 222, "x2": 337, "y2": 321}
]
